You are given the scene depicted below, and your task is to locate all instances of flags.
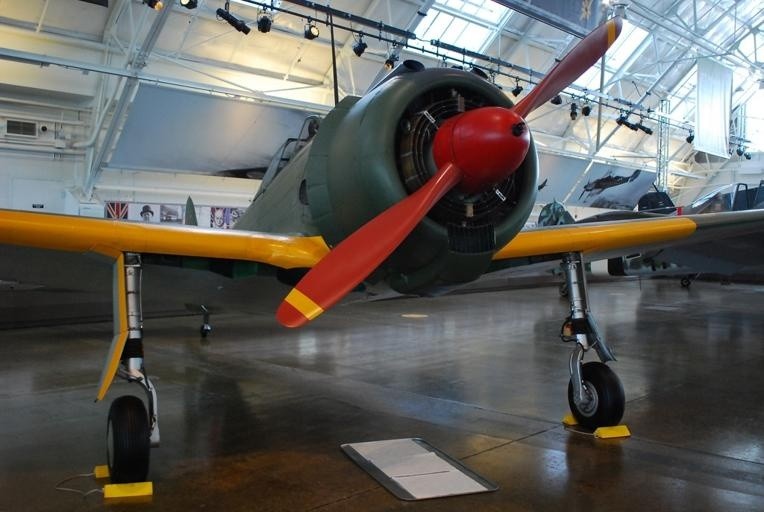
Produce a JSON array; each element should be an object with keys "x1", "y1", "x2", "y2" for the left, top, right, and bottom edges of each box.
[{"x1": 106, "y1": 202, "x2": 128, "y2": 220}]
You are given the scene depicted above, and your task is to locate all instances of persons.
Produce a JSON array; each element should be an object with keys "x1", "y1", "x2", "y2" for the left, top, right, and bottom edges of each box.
[
  {"x1": 139, "y1": 205, "x2": 154, "y2": 221},
  {"x1": 210, "y1": 208, "x2": 230, "y2": 229},
  {"x1": 584, "y1": 169, "x2": 641, "y2": 196}
]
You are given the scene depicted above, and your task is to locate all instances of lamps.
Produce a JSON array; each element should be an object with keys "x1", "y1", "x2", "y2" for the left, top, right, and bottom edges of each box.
[
  {"x1": 139, "y1": 0, "x2": 332, "y2": 41},
  {"x1": 551, "y1": 83, "x2": 656, "y2": 136},
  {"x1": 333, "y1": 9, "x2": 417, "y2": 71},
  {"x1": 429, "y1": 39, "x2": 544, "y2": 97}
]
